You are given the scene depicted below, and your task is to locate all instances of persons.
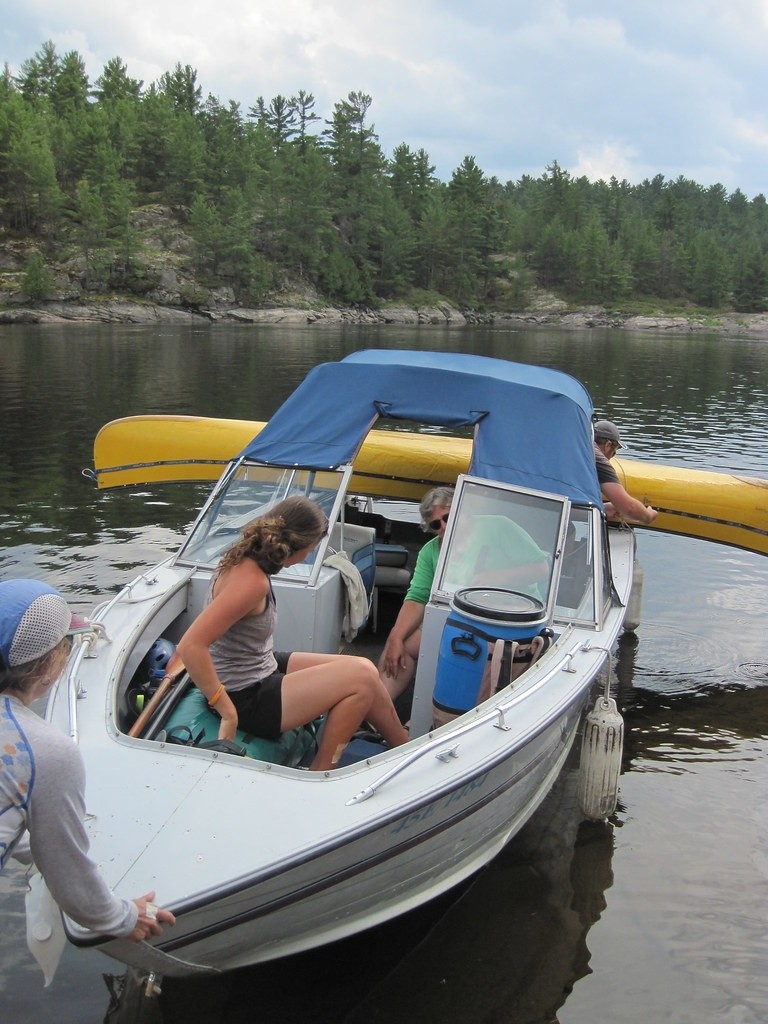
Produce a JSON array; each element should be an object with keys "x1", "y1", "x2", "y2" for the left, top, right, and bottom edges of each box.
[
  {"x1": 562, "y1": 421, "x2": 658, "y2": 576},
  {"x1": 0, "y1": 576, "x2": 179, "y2": 941},
  {"x1": 356, "y1": 485, "x2": 551, "y2": 748},
  {"x1": 175, "y1": 496, "x2": 410, "y2": 772}
]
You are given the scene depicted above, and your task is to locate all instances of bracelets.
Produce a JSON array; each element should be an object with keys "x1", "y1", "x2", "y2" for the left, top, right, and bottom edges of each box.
[{"x1": 207, "y1": 684, "x2": 225, "y2": 707}]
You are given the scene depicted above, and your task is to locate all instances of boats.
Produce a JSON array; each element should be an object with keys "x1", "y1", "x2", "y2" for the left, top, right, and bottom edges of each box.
[
  {"x1": 76, "y1": 412, "x2": 768, "y2": 560},
  {"x1": 33, "y1": 344, "x2": 636, "y2": 997}
]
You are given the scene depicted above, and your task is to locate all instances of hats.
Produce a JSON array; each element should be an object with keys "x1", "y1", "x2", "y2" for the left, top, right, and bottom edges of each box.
[
  {"x1": 593, "y1": 420, "x2": 629, "y2": 450},
  {"x1": 0, "y1": 579, "x2": 97, "y2": 668}
]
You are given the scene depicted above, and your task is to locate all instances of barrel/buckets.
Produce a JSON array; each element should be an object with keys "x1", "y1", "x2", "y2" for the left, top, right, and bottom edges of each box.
[{"x1": 430, "y1": 583, "x2": 551, "y2": 732}]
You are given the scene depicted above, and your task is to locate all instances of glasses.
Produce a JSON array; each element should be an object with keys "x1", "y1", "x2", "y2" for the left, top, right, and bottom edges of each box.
[{"x1": 428, "y1": 512, "x2": 451, "y2": 530}]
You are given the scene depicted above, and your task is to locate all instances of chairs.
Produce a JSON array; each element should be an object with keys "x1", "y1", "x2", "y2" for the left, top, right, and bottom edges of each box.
[{"x1": 325, "y1": 521, "x2": 379, "y2": 643}]
